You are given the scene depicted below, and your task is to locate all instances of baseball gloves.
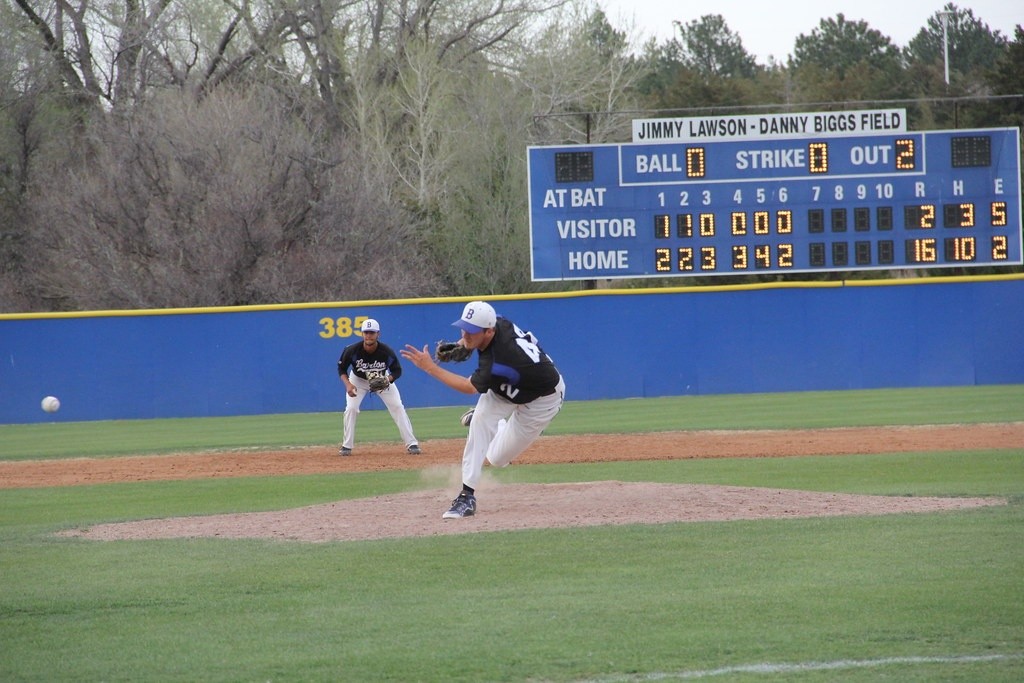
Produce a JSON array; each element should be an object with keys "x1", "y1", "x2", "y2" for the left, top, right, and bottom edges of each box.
[
  {"x1": 368, "y1": 377, "x2": 389, "y2": 394},
  {"x1": 434, "y1": 342, "x2": 473, "y2": 363}
]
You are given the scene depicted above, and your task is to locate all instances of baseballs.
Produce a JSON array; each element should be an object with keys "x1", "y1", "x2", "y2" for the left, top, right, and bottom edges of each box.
[{"x1": 41, "y1": 396, "x2": 59, "y2": 413}]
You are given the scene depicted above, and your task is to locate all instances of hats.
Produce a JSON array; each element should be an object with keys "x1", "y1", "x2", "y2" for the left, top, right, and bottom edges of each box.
[
  {"x1": 450, "y1": 300, "x2": 497, "y2": 334},
  {"x1": 361, "y1": 318, "x2": 381, "y2": 333}
]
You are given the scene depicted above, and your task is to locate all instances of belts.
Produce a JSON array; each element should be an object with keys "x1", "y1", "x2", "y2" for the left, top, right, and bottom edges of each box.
[{"x1": 541, "y1": 388, "x2": 557, "y2": 396}]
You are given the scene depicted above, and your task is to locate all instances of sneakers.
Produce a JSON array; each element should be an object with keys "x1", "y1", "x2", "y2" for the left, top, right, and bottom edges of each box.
[
  {"x1": 407, "y1": 445, "x2": 421, "y2": 454},
  {"x1": 338, "y1": 446, "x2": 351, "y2": 456},
  {"x1": 442, "y1": 490, "x2": 477, "y2": 522},
  {"x1": 460, "y1": 409, "x2": 475, "y2": 427}
]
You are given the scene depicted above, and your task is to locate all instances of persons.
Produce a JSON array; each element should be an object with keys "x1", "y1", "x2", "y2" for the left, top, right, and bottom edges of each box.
[
  {"x1": 399, "y1": 300, "x2": 566, "y2": 519},
  {"x1": 337, "y1": 319, "x2": 421, "y2": 454}
]
